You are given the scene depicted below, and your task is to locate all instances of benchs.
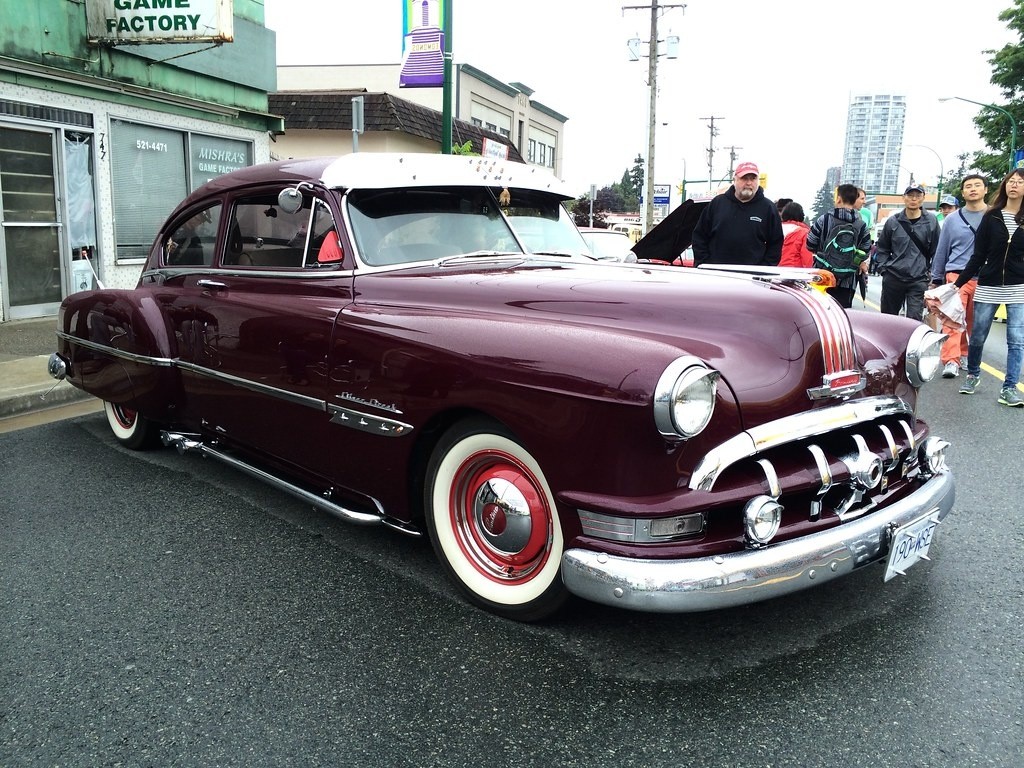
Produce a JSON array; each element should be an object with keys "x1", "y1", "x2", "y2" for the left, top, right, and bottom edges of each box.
[{"x1": 239, "y1": 248, "x2": 321, "y2": 264}]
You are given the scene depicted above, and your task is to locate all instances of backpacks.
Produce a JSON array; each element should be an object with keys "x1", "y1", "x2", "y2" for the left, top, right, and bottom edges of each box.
[{"x1": 816, "y1": 213, "x2": 868, "y2": 279}]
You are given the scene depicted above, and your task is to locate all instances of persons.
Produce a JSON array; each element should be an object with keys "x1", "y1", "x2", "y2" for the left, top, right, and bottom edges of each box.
[
  {"x1": 874, "y1": 168, "x2": 1024, "y2": 407},
  {"x1": 776, "y1": 184, "x2": 875, "y2": 308},
  {"x1": 691, "y1": 162, "x2": 784, "y2": 268}
]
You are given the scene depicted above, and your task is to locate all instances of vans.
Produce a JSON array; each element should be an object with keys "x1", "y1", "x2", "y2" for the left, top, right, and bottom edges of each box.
[{"x1": 613, "y1": 222, "x2": 641, "y2": 244}]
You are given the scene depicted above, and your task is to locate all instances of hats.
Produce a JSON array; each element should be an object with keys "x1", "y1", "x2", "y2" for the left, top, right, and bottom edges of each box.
[
  {"x1": 939, "y1": 196, "x2": 959, "y2": 207},
  {"x1": 735, "y1": 162, "x2": 759, "y2": 178},
  {"x1": 904, "y1": 184, "x2": 925, "y2": 195}
]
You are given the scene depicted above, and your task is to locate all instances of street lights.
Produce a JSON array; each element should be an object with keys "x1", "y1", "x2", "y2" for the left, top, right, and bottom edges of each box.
[
  {"x1": 938, "y1": 95, "x2": 1015, "y2": 173},
  {"x1": 896, "y1": 144, "x2": 943, "y2": 211},
  {"x1": 892, "y1": 163, "x2": 913, "y2": 186}
]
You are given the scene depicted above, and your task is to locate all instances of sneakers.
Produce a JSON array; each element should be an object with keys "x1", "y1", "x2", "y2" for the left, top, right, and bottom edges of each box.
[
  {"x1": 942, "y1": 361, "x2": 959, "y2": 377},
  {"x1": 959, "y1": 357, "x2": 968, "y2": 371},
  {"x1": 997, "y1": 388, "x2": 1024, "y2": 407},
  {"x1": 959, "y1": 374, "x2": 980, "y2": 395}
]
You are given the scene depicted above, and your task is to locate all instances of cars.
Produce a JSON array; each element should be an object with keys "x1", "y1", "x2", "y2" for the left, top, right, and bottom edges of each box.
[
  {"x1": 492, "y1": 227, "x2": 694, "y2": 267},
  {"x1": 48, "y1": 152, "x2": 956, "y2": 618}
]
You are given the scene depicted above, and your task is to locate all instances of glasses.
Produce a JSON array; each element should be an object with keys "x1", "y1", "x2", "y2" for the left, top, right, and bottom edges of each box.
[{"x1": 1006, "y1": 180, "x2": 1024, "y2": 186}]
